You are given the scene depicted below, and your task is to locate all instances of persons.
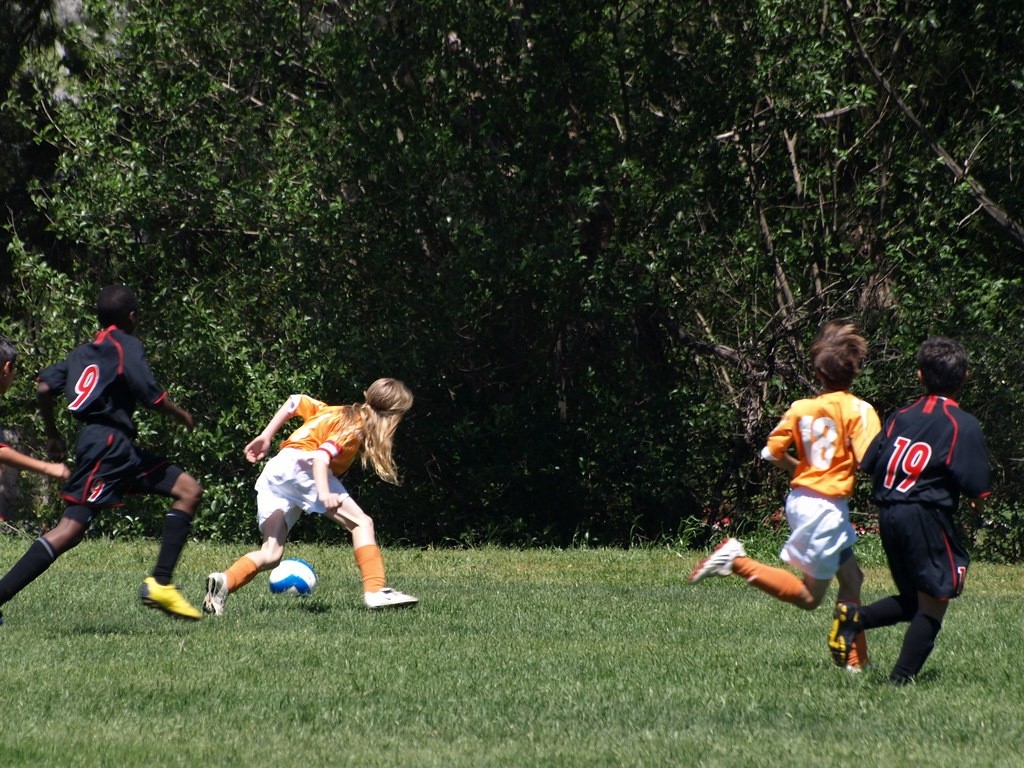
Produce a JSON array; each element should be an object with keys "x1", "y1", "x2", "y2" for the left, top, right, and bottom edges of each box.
[
  {"x1": 0, "y1": 336, "x2": 71, "y2": 479},
  {"x1": 0, "y1": 285, "x2": 203, "y2": 621},
  {"x1": 827, "y1": 338, "x2": 990, "y2": 691},
  {"x1": 689, "y1": 323, "x2": 882, "y2": 671},
  {"x1": 202, "y1": 377, "x2": 420, "y2": 616}
]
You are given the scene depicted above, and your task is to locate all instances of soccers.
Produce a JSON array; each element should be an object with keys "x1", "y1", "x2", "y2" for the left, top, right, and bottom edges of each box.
[{"x1": 268, "y1": 557, "x2": 318, "y2": 598}]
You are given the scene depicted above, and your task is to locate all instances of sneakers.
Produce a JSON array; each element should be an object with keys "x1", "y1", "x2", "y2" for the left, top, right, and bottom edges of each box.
[
  {"x1": 827, "y1": 598, "x2": 861, "y2": 667},
  {"x1": 846, "y1": 662, "x2": 874, "y2": 673},
  {"x1": 140, "y1": 577, "x2": 202, "y2": 620},
  {"x1": 690, "y1": 538, "x2": 747, "y2": 584},
  {"x1": 364, "y1": 587, "x2": 419, "y2": 609},
  {"x1": 203, "y1": 572, "x2": 229, "y2": 616},
  {"x1": 889, "y1": 670, "x2": 919, "y2": 687}
]
